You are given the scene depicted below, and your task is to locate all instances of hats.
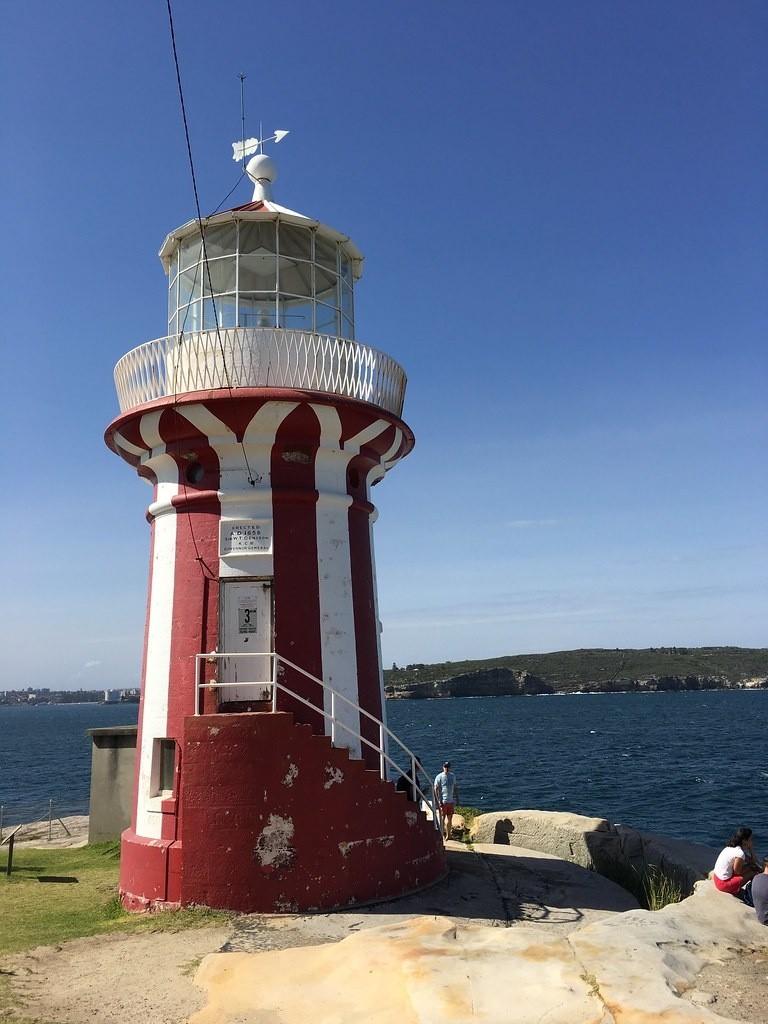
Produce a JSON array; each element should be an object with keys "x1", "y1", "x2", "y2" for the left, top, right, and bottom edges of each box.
[{"x1": 443, "y1": 762, "x2": 451, "y2": 767}]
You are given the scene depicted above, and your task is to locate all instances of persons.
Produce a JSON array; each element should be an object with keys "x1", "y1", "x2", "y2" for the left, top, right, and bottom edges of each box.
[
  {"x1": 753, "y1": 854, "x2": 768, "y2": 927},
  {"x1": 712, "y1": 826, "x2": 763, "y2": 894},
  {"x1": 397, "y1": 756, "x2": 430, "y2": 803},
  {"x1": 433, "y1": 762, "x2": 460, "y2": 841}
]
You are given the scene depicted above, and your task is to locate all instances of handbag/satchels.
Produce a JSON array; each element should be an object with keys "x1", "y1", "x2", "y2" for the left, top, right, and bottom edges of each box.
[{"x1": 740, "y1": 880, "x2": 754, "y2": 907}]
